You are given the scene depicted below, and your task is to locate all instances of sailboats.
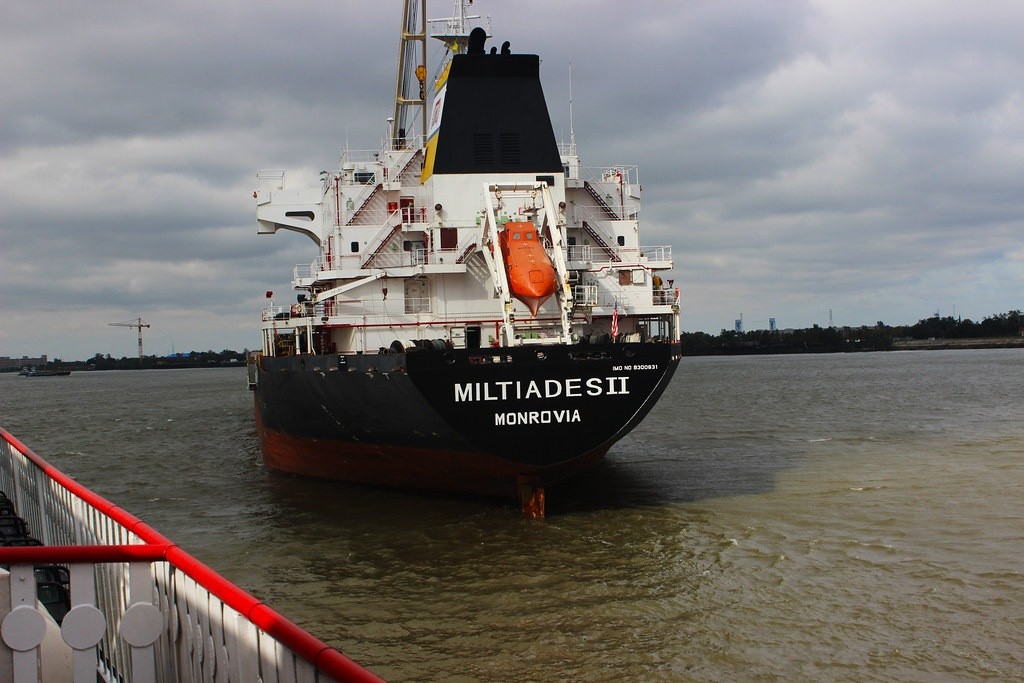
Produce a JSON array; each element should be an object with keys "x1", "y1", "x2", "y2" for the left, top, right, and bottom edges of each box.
[{"x1": 247, "y1": 1, "x2": 683, "y2": 521}]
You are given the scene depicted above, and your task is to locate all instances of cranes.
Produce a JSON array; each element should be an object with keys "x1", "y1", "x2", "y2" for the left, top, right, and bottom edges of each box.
[{"x1": 108, "y1": 318, "x2": 150, "y2": 358}]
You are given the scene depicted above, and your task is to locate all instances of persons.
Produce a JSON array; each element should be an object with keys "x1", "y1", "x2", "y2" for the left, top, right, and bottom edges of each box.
[{"x1": 547, "y1": 202, "x2": 567, "y2": 249}]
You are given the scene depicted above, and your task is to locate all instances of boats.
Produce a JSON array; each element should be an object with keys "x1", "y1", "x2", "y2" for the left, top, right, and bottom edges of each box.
[
  {"x1": 17, "y1": 370, "x2": 29, "y2": 376},
  {"x1": 27, "y1": 369, "x2": 71, "y2": 377}
]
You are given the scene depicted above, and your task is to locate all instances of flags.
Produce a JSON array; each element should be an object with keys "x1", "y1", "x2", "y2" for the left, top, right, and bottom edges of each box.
[{"x1": 611, "y1": 300, "x2": 619, "y2": 337}]
